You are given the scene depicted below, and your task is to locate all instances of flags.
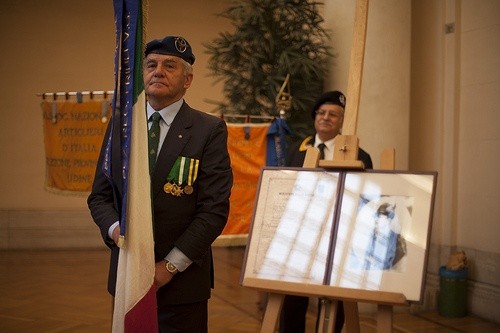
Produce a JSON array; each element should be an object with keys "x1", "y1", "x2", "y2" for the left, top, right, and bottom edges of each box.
[{"x1": 107, "y1": 0, "x2": 160, "y2": 333}]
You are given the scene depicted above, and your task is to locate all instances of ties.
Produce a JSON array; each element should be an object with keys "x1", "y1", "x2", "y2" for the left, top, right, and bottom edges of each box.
[
  {"x1": 318, "y1": 143, "x2": 326, "y2": 160},
  {"x1": 148, "y1": 113, "x2": 161, "y2": 175}
]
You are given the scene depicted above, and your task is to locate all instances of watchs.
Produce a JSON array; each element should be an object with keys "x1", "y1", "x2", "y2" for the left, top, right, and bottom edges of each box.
[{"x1": 163, "y1": 260, "x2": 179, "y2": 276}]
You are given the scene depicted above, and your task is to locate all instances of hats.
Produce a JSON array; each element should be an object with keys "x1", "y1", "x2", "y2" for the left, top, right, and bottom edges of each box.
[
  {"x1": 312, "y1": 91, "x2": 346, "y2": 119},
  {"x1": 145, "y1": 36, "x2": 195, "y2": 64}
]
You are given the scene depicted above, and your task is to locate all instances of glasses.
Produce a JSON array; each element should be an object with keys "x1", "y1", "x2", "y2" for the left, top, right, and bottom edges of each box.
[{"x1": 315, "y1": 110, "x2": 342, "y2": 117}]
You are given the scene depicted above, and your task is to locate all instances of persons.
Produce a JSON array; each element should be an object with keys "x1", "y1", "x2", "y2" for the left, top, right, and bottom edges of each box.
[
  {"x1": 86, "y1": 35, "x2": 233, "y2": 333},
  {"x1": 277, "y1": 91, "x2": 373, "y2": 333}
]
